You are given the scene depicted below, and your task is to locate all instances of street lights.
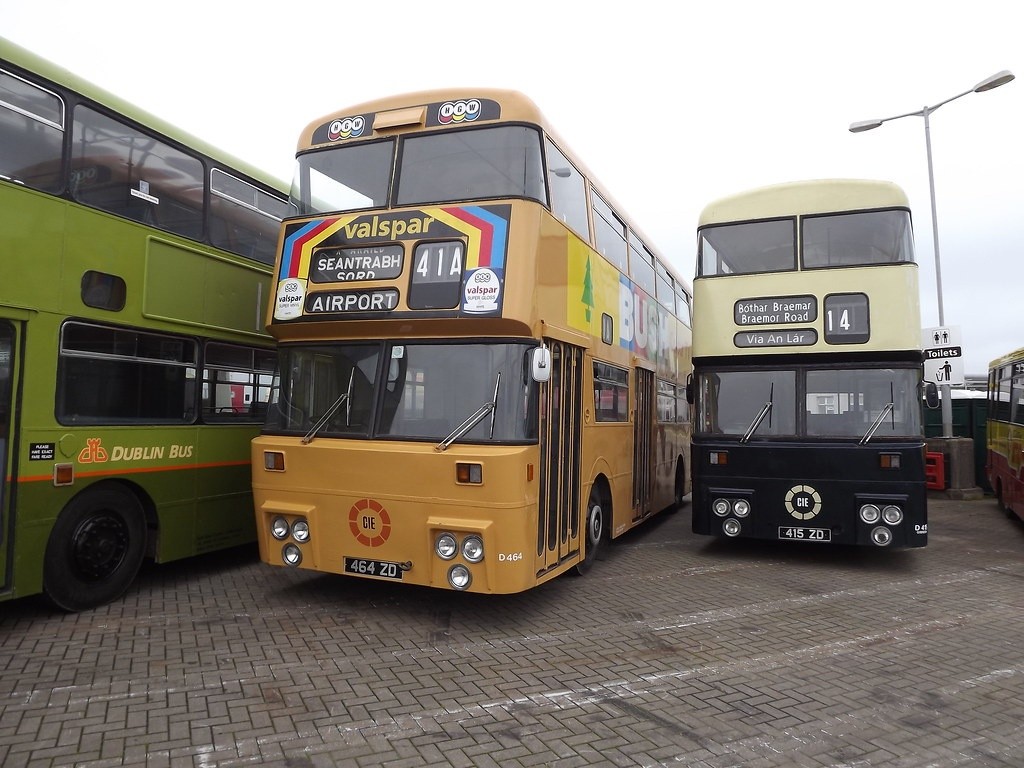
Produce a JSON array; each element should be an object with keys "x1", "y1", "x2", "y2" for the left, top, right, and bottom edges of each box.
[{"x1": 846, "y1": 67, "x2": 1016, "y2": 436}]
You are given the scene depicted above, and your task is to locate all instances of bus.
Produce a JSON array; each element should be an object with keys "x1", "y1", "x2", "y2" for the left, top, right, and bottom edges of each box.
[
  {"x1": 684, "y1": 178, "x2": 931, "y2": 560},
  {"x1": 0, "y1": 33, "x2": 339, "y2": 615},
  {"x1": 985, "y1": 346, "x2": 1024, "y2": 538},
  {"x1": 245, "y1": 88, "x2": 695, "y2": 596}
]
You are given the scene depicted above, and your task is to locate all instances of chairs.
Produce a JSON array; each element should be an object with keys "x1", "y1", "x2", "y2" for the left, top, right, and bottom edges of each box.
[{"x1": 248, "y1": 402, "x2": 269, "y2": 416}]
